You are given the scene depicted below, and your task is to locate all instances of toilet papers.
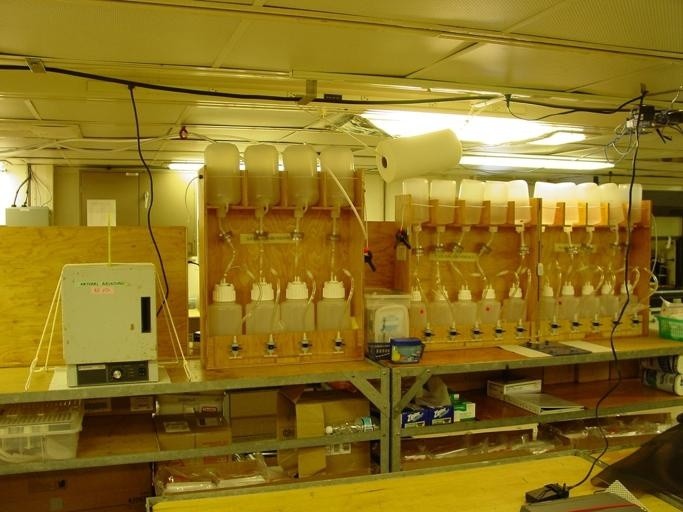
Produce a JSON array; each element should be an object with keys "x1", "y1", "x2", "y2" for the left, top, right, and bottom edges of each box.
[{"x1": 375, "y1": 127, "x2": 464, "y2": 181}]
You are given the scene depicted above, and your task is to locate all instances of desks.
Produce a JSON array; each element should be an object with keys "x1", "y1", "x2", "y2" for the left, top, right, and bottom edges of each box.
[
  {"x1": 146, "y1": 447, "x2": 683, "y2": 512},
  {"x1": 0, "y1": 355, "x2": 389, "y2": 475},
  {"x1": 391, "y1": 331, "x2": 683, "y2": 471}
]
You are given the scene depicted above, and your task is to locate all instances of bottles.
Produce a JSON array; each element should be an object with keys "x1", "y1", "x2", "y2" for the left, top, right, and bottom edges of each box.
[{"x1": 325, "y1": 416, "x2": 379, "y2": 433}]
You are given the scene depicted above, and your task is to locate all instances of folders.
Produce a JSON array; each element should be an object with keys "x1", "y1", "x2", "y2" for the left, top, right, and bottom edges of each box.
[{"x1": 505, "y1": 393, "x2": 584, "y2": 415}]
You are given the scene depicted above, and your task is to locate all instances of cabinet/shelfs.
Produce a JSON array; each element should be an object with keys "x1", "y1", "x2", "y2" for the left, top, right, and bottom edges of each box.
[
  {"x1": 79, "y1": 170, "x2": 198, "y2": 257},
  {"x1": 197, "y1": 166, "x2": 365, "y2": 371},
  {"x1": 395, "y1": 194, "x2": 540, "y2": 352},
  {"x1": 540, "y1": 199, "x2": 652, "y2": 341}
]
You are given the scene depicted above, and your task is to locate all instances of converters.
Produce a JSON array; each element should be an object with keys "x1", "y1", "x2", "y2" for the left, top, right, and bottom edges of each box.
[{"x1": 524, "y1": 482, "x2": 570, "y2": 503}]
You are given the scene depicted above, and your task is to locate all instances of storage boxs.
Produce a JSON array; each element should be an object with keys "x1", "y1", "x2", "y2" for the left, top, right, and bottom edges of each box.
[
  {"x1": 0, "y1": 463, "x2": 154, "y2": 512},
  {"x1": 155, "y1": 384, "x2": 373, "y2": 492}
]
